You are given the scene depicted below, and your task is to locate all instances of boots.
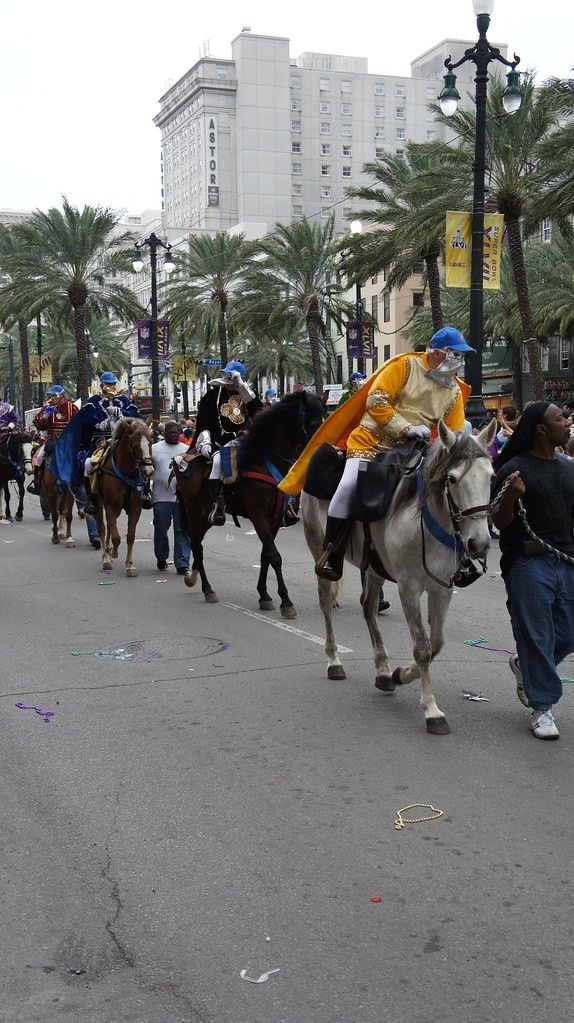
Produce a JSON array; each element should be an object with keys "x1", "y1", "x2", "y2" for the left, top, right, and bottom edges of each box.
[
  {"x1": 83, "y1": 475, "x2": 94, "y2": 510},
  {"x1": 322, "y1": 515, "x2": 349, "y2": 580},
  {"x1": 200, "y1": 479, "x2": 226, "y2": 526},
  {"x1": 27, "y1": 465, "x2": 40, "y2": 493},
  {"x1": 279, "y1": 501, "x2": 300, "y2": 527}
]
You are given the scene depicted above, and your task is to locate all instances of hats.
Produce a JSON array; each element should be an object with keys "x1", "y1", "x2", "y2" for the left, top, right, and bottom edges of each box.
[
  {"x1": 348, "y1": 372, "x2": 367, "y2": 382},
  {"x1": 265, "y1": 389, "x2": 276, "y2": 396},
  {"x1": 100, "y1": 372, "x2": 118, "y2": 382},
  {"x1": 430, "y1": 327, "x2": 477, "y2": 355},
  {"x1": 47, "y1": 385, "x2": 64, "y2": 394},
  {"x1": 220, "y1": 361, "x2": 246, "y2": 376}
]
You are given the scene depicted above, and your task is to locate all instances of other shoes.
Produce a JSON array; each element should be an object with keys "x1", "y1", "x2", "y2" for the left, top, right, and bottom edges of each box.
[
  {"x1": 378, "y1": 599, "x2": 390, "y2": 612},
  {"x1": 177, "y1": 567, "x2": 188, "y2": 574},
  {"x1": 157, "y1": 560, "x2": 168, "y2": 570},
  {"x1": 92, "y1": 538, "x2": 101, "y2": 549}
]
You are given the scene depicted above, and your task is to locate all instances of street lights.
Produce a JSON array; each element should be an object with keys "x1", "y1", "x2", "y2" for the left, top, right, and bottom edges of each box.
[
  {"x1": 436, "y1": 0, "x2": 525, "y2": 427},
  {"x1": 132, "y1": 231, "x2": 175, "y2": 427},
  {"x1": 337, "y1": 220, "x2": 381, "y2": 375}
]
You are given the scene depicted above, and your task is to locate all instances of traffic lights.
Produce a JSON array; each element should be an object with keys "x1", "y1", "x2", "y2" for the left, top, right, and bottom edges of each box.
[{"x1": 173, "y1": 383, "x2": 181, "y2": 403}]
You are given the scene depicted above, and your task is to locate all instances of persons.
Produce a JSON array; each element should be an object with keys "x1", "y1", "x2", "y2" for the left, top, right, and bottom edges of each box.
[
  {"x1": 315, "y1": 326, "x2": 485, "y2": 588},
  {"x1": 27, "y1": 385, "x2": 80, "y2": 494},
  {"x1": 168, "y1": 361, "x2": 264, "y2": 526},
  {"x1": 75, "y1": 500, "x2": 101, "y2": 550},
  {"x1": 150, "y1": 418, "x2": 195, "y2": 446},
  {"x1": 262, "y1": 389, "x2": 278, "y2": 409},
  {"x1": 0, "y1": 397, "x2": 20, "y2": 435},
  {"x1": 491, "y1": 402, "x2": 574, "y2": 739},
  {"x1": 152, "y1": 423, "x2": 190, "y2": 576},
  {"x1": 49, "y1": 372, "x2": 153, "y2": 515},
  {"x1": 473, "y1": 405, "x2": 574, "y2": 538},
  {"x1": 336, "y1": 372, "x2": 367, "y2": 408}
]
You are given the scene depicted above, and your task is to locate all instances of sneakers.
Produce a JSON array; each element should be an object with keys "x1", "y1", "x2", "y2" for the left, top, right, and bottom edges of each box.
[
  {"x1": 528, "y1": 709, "x2": 560, "y2": 739},
  {"x1": 509, "y1": 654, "x2": 530, "y2": 707}
]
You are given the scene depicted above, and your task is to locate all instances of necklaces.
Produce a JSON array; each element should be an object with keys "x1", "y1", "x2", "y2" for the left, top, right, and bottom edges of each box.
[{"x1": 227, "y1": 389, "x2": 243, "y2": 415}]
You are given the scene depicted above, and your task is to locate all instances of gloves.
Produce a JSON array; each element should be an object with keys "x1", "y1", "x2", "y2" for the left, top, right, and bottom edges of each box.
[
  {"x1": 201, "y1": 444, "x2": 211, "y2": 459},
  {"x1": 45, "y1": 406, "x2": 57, "y2": 414},
  {"x1": 106, "y1": 406, "x2": 116, "y2": 415},
  {"x1": 405, "y1": 424, "x2": 431, "y2": 439},
  {"x1": 230, "y1": 369, "x2": 244, "y2": 386}
]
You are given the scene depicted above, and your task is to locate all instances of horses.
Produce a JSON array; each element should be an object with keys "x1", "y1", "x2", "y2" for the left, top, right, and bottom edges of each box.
[
  {"x1": 298, "y1": 418, "x2": 498, "y2": 736},
  {"x1": 85, "y1": 415, "x2": 154, "y2": 577},
  {"x1": 0, "y1": 430, "x2": 36, "y2": 524},
  {"x1": 34, "y1": 443, "x2": 77, "y2": 548},
  {"x1": 171, "y1": 389, "x2": 329, "y2": 618}
]
